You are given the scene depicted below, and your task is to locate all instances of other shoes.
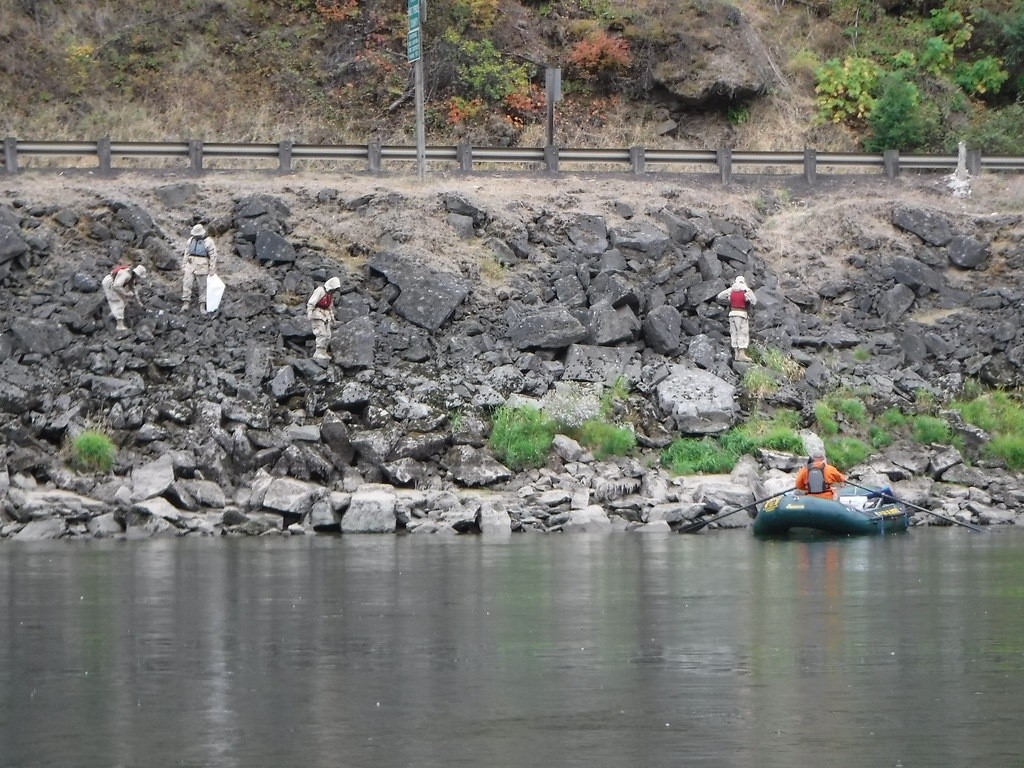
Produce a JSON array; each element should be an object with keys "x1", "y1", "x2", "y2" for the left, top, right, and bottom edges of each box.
[
  {"x1": 199, "y1": 303, "x2": 208, "y2": 315},
  {"x1": 313, "y1": 352, "x2": 328, "y2": 359},
  {"x1": 116, "y1": 326, "x2": 127, "y2": 330},
  {"x1": 324, "y1": 352, "x2": 332, "y2": 360},
  {"x1": 738, "y1": 356, "x2": 753, "y2": 362},
  {"x1": 181, "y1": 302, "x2": 190, "y2": 312},
  {"x1": 734, "y1": 356, "x2": 749, "y2": 361}
]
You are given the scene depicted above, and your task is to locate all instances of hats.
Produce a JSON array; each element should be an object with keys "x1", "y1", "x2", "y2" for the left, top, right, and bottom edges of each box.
[
  {"x1": 190, "y1": 224, "x2": 206, "y2": 236},
  {"x1": 132, "y1": 264, "x2": 148, "y2": 278}
]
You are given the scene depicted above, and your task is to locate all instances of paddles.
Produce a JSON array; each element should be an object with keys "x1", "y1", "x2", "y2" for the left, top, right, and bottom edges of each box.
[
  {"x1": 677, "y1": 487, "x2": 798, "y2": 534},
  {"x1": 843, "y1": 480, "x2": 982, "y2": 532}
]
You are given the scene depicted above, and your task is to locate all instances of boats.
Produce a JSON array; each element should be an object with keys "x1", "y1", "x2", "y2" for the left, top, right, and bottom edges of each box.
[{"x1": 752, "y1": 484, "x2": 909, "y2": 539}]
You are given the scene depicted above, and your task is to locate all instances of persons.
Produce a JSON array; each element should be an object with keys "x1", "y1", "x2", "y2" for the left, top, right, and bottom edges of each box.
[
  {"x1": 795, "y1": 451, "x2": 846, "y2": 500},
  {"x1": 717, "y1": 276, "x2": 757, "y2": 363},
  {"x1": 307, "y1": 277, "x2": 340, "y2": 360},
  {"x1": 102, "y1": 264, "x2": 146, "y2": 332},
  {"x1": 179, "y1": 224, "x2": 217, "y2": 315}
]
